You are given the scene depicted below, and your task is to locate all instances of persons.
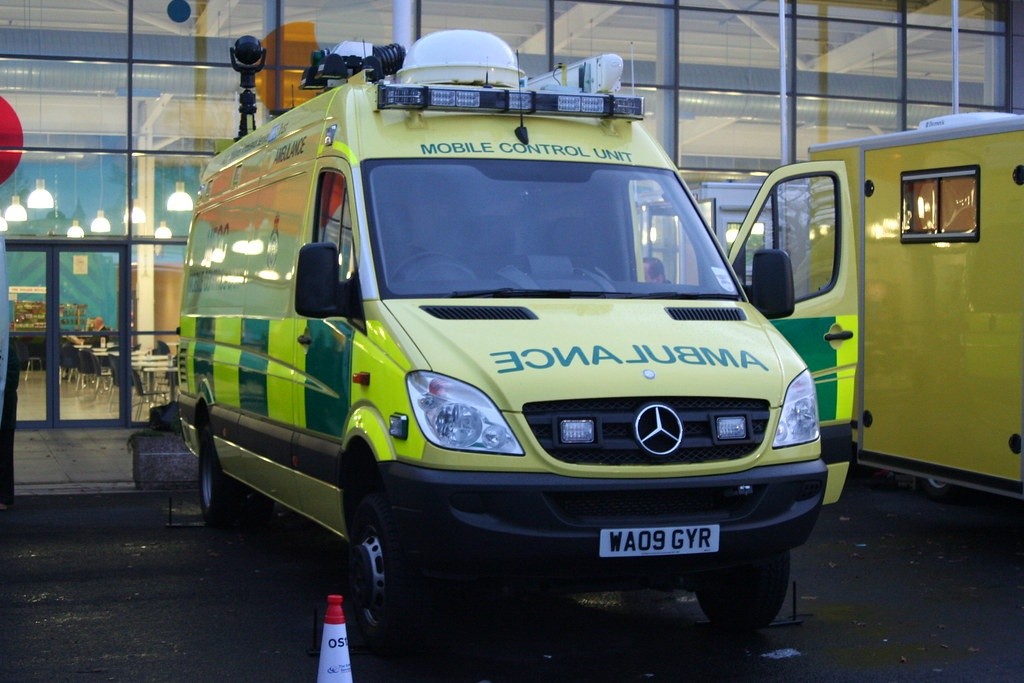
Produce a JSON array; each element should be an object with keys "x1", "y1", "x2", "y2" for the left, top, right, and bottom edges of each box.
[
  {"x1": 641, "y1": 258, "x2": 669, "y2": 286},
  {"x1": 80, "y1": 318, "x2": 108, "y2": 346}
]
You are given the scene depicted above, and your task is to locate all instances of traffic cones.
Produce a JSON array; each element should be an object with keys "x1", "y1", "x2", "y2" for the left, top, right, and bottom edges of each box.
[{"x1": 314, "y1": 593, "x2": 354, "y2": 683}]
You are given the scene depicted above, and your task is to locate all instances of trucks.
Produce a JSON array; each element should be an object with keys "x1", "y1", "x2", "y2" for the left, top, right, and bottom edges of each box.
[{"x1": 801, "y1": 111, "x2": 1023, "y2": 502}]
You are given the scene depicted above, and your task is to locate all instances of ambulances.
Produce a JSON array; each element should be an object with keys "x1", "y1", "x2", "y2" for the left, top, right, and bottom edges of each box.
[{"x1": 173, "y1": 26, "x2": 860, "y2": 656}]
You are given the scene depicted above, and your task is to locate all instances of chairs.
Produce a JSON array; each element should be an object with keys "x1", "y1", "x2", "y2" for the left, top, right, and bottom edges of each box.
[{"x1": 14, "y1": 338, "x2": 170, "y2": 422}]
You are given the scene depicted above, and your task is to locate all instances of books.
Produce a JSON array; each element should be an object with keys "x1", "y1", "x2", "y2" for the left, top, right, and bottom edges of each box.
[{"x1": 63, "y1": 336, "x2": 81, "y2": 345}]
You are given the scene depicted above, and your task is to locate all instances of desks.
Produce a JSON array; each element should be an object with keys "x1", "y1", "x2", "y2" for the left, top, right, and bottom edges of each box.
[
  {"x1": 74, "y1": 344, "x2": 93, "y2": 350},
  {"x1": 130, "y1": 361, "x2": 172, "y2": 368},
  {"x1": 93, "y1": 352, "x2": 109, "y2": 357},
  {"x1": 131, "y1": 355, "x2": 169, "y2": 361},
  {"x1": 144, "y1": 367, "x2": 186, "y2": 402}
]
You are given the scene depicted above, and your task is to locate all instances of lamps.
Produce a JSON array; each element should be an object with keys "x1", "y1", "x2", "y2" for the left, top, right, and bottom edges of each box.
[
  {"x1": 67, "y1": 123, "x2": 84, "y2": 238},
  {"x1": 28, "y1": 66, "x2": 54, "y2": 208},
  {"x1": 90, "y1": 92, "x2": 111, "y2": 234},
  {"x1": 6, "y1": 170, "x2": 24, "y2": 223},
  {"x1": 156, "y1": 165, "x2": 173, "y2": 239},
  {"x1": 168, "y1": 101, "x2": 194, "y2": 212}
]
